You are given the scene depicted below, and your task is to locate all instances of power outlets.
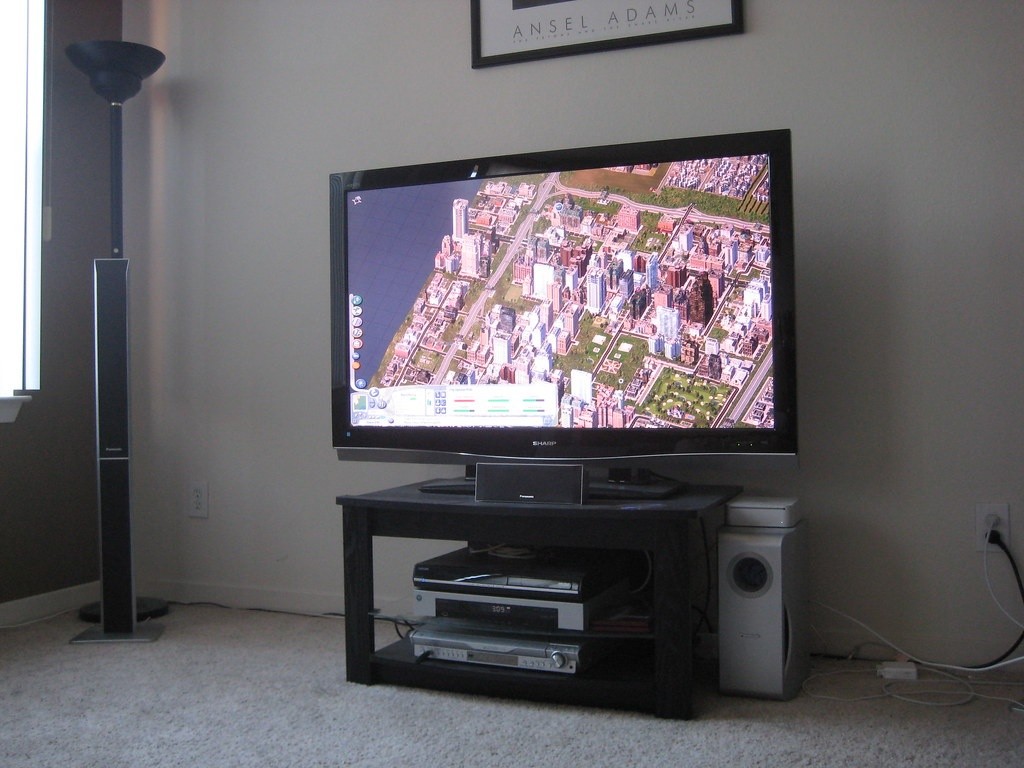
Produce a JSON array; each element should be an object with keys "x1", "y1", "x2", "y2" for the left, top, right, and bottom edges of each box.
[
  {"x1": 187, "y1": 480, "x2": 209, "y2": 518},
  {"x1": 975, "y1": 501, "x2": 1011, "y2": 552}
]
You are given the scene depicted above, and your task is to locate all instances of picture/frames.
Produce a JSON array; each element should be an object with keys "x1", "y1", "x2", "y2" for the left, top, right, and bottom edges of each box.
[{"x1": 469, "y1": 0, "x2": 744, "y2": 70}]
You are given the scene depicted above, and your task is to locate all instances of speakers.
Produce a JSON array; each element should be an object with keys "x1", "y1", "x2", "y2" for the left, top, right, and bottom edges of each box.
[
  {"x1": 718, "y1": 518, "x2": 809, "y2": 700},
  {"x1": 475, "y1": 462, "x2": 589, "y2": 506},
  {"x1": 94, "y1": 257, "x2": 138, "y2": 634}
]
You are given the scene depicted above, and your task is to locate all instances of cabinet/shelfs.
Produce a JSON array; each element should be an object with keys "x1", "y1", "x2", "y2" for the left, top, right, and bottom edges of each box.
[{"x1": 335, "y1": 478, "x2": 745, "y2": 720}]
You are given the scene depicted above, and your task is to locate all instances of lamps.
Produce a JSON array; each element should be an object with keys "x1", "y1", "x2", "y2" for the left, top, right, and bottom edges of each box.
[{"x1": 62, "y1": 38, "x2": 172, "y2": 623}]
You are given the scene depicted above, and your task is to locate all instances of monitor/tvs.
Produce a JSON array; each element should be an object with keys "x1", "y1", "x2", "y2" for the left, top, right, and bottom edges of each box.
[{"x1": 330, "y1": 128, "x2": 794, "y2": 498}]
